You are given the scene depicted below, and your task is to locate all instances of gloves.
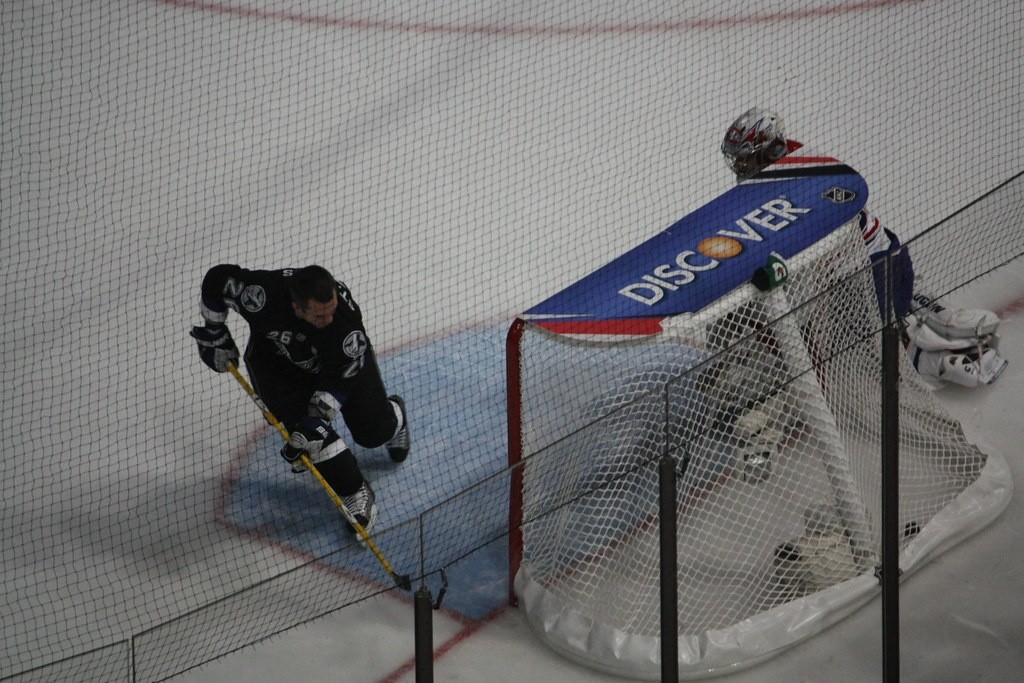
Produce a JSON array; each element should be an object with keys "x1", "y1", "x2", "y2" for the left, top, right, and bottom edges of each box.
[
  {"x1": 280, "y1": 416, "x2": 332, "y2": 473},
  {"x1": 189, "y1": 325, "x2": 239, "y2": 373}
]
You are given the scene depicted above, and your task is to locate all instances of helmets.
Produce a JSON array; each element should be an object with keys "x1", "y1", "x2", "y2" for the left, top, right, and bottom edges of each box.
[{"x1": 720, "y1": 106, "x2": 788, "y2": 185}]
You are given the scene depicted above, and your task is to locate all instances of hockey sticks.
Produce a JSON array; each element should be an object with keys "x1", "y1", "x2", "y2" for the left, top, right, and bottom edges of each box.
[{"x1": 228, "y1": 360, "x2": 413, "y2": 593}]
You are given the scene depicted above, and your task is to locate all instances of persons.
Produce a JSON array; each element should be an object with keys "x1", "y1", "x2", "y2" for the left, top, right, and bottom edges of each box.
[
  {"x1": 708, "y1": 107, "x2": 1008, "y2": 444},
  {"x1": 189, "y1": 265, "x2": 410, "y2": 548}
]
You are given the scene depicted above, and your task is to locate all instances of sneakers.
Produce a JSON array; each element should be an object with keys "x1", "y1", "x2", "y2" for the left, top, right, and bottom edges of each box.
[
  {"x1": 386, "y1": 395, "x2": 410, "y2": 462},
  {"x1": 940, "y1": 345, "x2": 1009, "y2": 389},
  {"x1": 343, "y1": 478, "x2": 380, "y2": 548}
]
